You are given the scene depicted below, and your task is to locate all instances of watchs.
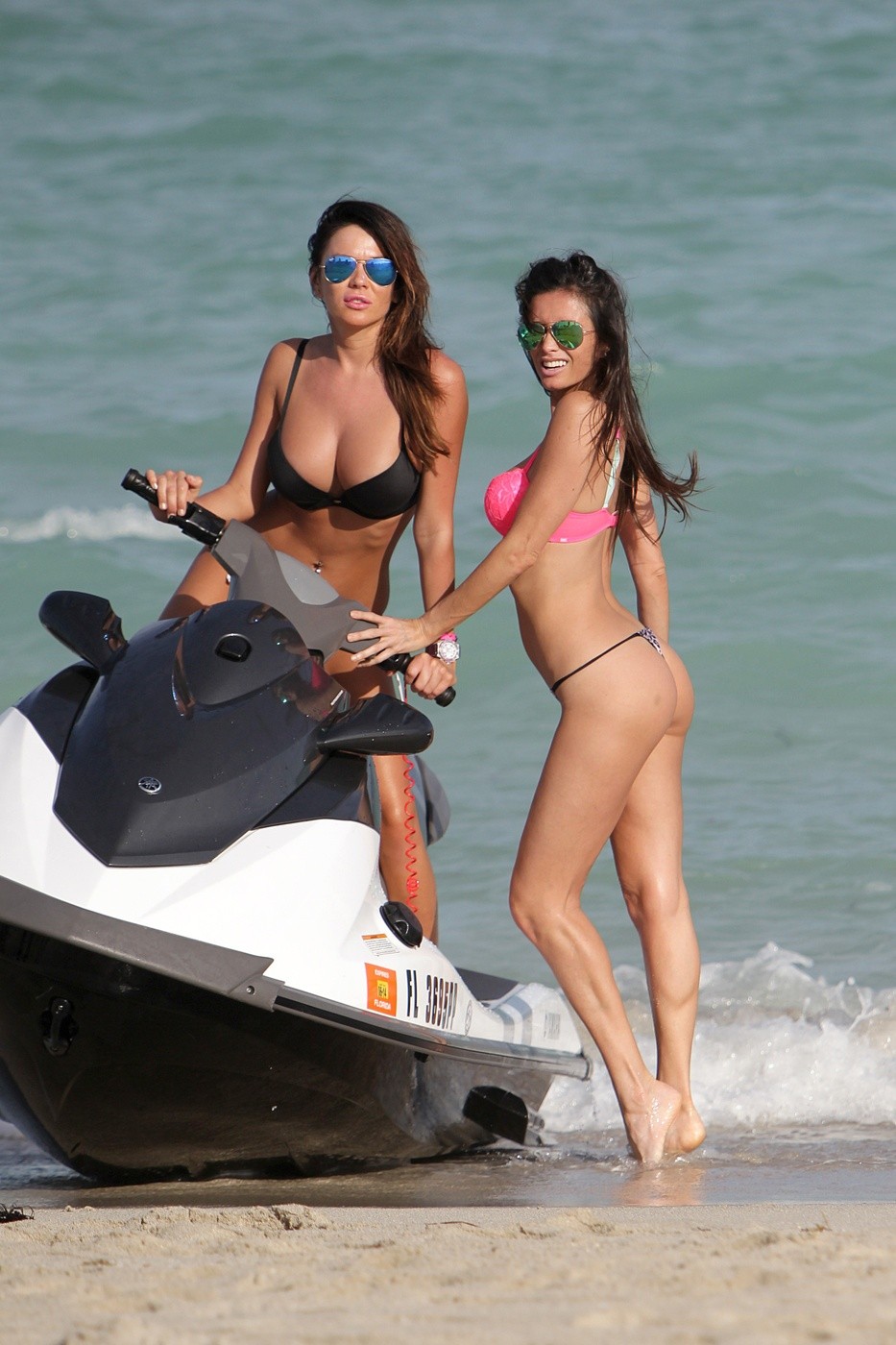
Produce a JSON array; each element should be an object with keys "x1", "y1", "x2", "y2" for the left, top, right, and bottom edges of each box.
[{"x1": 424, "y1": 636, "x2": 464, "y2": 664}]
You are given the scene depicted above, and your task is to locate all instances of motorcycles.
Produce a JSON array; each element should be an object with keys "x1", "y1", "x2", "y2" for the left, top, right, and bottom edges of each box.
[{"x1": 1, "y1": 467, "x2": 593, "y2": 1182}]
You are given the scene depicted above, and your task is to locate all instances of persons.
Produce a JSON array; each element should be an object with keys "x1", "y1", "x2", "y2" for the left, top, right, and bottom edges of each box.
[
  {"x1": 149, "y1": 196, "x2": 474, "y2": 947},
  {"x1": 344, "y1": 249, "x2": 709, "y2": 1168}
]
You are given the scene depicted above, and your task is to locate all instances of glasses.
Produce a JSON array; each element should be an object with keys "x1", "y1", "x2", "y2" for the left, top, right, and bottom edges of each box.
[
  {"x1": 517, "y1": 320, "x2": 596, "y2": 350},
  {"x1": 318, "y1": 254, "x2": 399, "y2": 287}
]
